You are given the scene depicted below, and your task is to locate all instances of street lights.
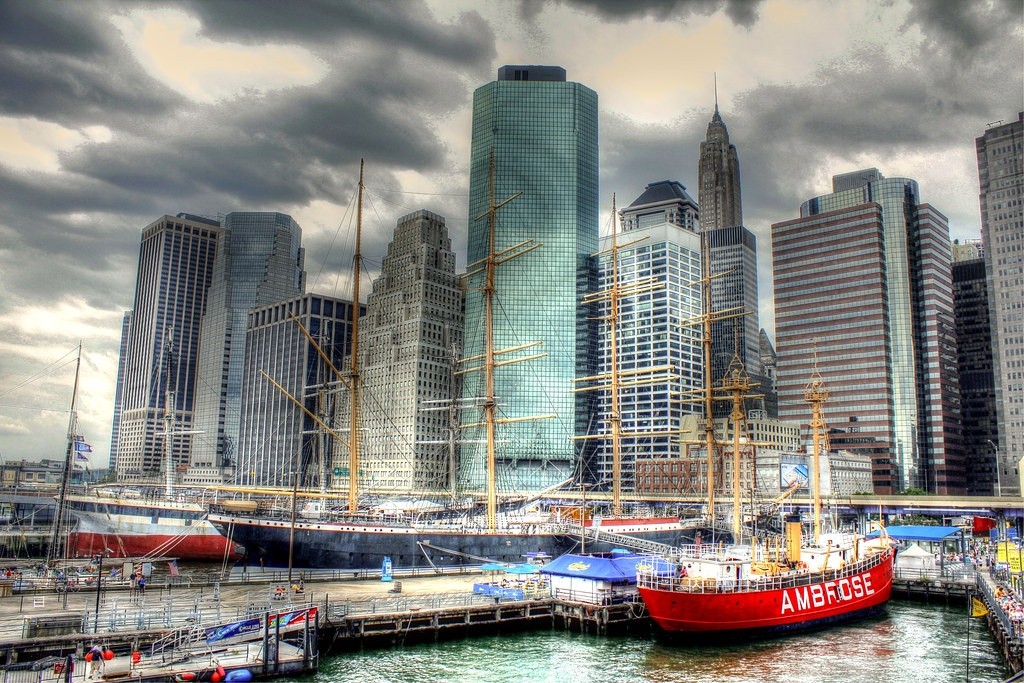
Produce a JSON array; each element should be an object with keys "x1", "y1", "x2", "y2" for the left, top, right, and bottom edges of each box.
[{"x1": 988, "y1": 440, "x2": 1001, "y2": 497}]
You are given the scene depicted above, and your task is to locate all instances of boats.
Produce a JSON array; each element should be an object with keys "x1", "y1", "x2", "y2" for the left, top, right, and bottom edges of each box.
[
  {"x1": 207, "y1": 146, "x2": 801, "y2": 569},
  {"x1": 635, "y1": 337, "x2": 898, "y2": 642},
  {"x1": 50, "y1": 490, "x2": 246, "y2": 565}
]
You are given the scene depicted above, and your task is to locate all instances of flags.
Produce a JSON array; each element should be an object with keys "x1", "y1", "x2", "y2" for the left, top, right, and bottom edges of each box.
[
  {"x1": 167, "y1": 561, "x2": 179, "y2": 576},
  {"x1": 74, "y1": 436, "x2": 92, "y2": 462}
]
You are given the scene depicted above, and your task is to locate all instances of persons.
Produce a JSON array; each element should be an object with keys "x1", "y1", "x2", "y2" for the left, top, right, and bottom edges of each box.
[
  {"x1": 57, "y1": 567, "x2": 117, "y2": 586},
  {"x1": 677, "y1": 566, "x2": 687, "y2": 585},
  {"x1": 130, "y1": 572, "x2": 145, "y2": 595},
  {"x1": 993, "y1": 584, "x2": 1024, "y2": 636},
  {"x1": 34, "y1": 561, "x2": 49, "y2": 577},
  {"x1": 949, "y1": 542, "x2": 995, "y2": 571},
  {"x1": 7, "y1": 568, "x2": 20, "y2": 581},
  {"x1": 88, "y1": 641, "x2": 105, "y2": 679},
  {"x1": 274, "y1": 575, "x2": 305, "y2": 599},
  {"x1": 498, "y1": 579, "x2": 507, "y2": 587}
]
